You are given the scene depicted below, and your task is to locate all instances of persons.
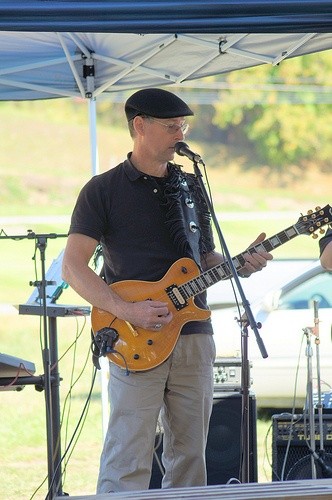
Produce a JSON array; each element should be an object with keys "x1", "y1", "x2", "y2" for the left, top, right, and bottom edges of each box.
[
  {"x1": 318, "y1": 225, "x2": 332, "y2": 272},
  {"x1": 62, "y1": 88, "x2": 273, "y2": 495}
]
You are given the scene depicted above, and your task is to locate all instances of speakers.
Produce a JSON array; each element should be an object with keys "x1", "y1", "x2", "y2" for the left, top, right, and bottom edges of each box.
[
  {"x1": 272, "y1": 414, "x2": 332, "y2": 482},
  {"x1": 148, "y1": 391, "x2": 259, "y2": 489}
]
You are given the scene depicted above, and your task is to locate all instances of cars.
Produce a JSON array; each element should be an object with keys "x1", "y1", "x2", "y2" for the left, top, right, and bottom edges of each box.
[{"x1": 223, "y1": 265, "x2": 331, "y2": 364}]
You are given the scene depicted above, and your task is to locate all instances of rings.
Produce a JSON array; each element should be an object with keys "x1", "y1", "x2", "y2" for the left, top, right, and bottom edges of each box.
[{"x1": 154, "y1": 323, "x2": 161, "y2": 328}]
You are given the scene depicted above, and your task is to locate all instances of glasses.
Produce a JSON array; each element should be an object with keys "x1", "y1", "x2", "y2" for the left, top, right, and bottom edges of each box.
[{"x1": 146, "y1": 117, "x2": 189, "y2": 134}]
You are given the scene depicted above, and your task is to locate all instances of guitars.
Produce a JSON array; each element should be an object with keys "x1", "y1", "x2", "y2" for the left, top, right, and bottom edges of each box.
[{"x1": 91, "y1": 204, "x2": 332, "y2": 373}]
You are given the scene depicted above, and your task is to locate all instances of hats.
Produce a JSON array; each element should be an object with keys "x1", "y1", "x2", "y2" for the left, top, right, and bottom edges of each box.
[{"x1": 125, "y1": 89, "x2": 195, "y2": 122}]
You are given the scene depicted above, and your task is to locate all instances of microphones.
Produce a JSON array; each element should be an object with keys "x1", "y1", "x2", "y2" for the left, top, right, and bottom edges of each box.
[
  {"x1": 309, "y1": 448, "x2": 332, "y2": 479},
  {"x1": 175, "y1": 142, "x2": 203, "y2": 164}
]
